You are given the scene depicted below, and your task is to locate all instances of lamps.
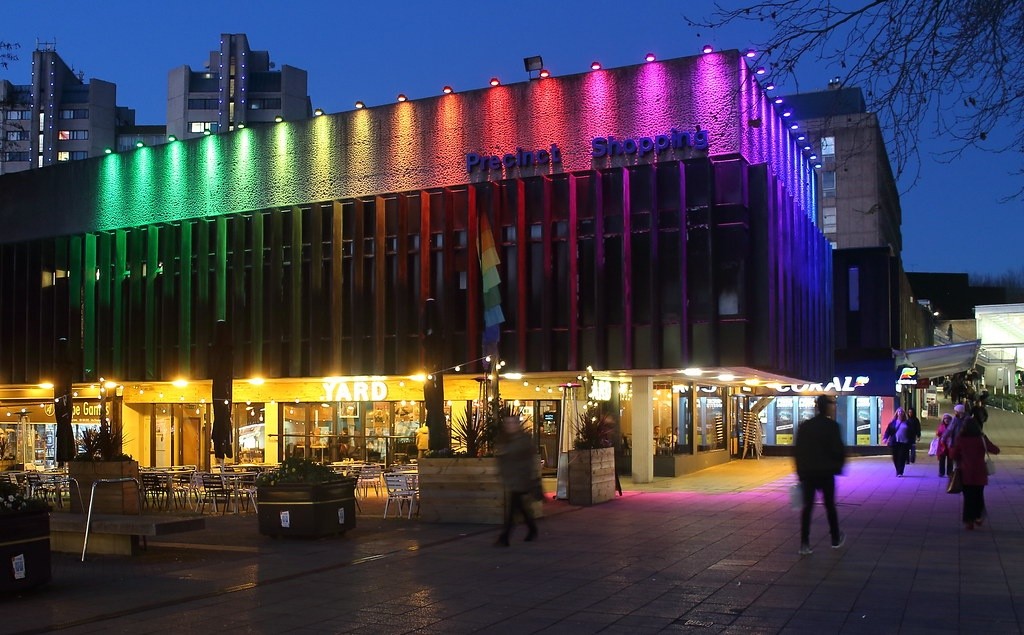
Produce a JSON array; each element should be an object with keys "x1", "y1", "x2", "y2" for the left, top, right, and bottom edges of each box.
[
  {"x1": 743, "y1": 48, "x2": 822, "y2": 170},
  {"x1": 104, "y1": 78, "x2": 501, "y2": 154},
  {"x1": 524, "y1": 44, "x2": 714, "y2": 81}
]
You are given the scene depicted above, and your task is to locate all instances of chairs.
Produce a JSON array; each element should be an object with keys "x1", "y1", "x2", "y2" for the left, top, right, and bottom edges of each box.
[
  {"x1": 0, "y1": 468, "x2": 69, "y2": 511},
  {"x1": 138, "y1": 461, "x2": 266, "y2": 515},
  {"x1": 313, "y1": 457, "x2": 418, "y2": 519}
]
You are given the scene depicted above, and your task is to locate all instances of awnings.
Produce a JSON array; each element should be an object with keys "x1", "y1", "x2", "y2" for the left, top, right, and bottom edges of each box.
[{"x1": 906, "y1": 338, "x2": 981, "y2": 380}]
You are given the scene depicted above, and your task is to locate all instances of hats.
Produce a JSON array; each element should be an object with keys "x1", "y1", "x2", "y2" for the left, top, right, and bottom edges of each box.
[{"x1": 954, "y1": 405, "x2": 966, "y2": 413}]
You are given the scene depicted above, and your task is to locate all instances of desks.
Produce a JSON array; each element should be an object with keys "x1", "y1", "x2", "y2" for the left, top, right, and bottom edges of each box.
[
  {"x1": 327, "y1": 464, "x2": 364, "y2": 475},
  {"x1": 139, "y1": 464, "x2": 274, "y2": 515},
  {"x1": 333, "y1": 461, "x2": 365, "y2": 464},
  {"x1": 26, "y1": 472, "x2": 65, "y2": 502},
  {"x1": 405, "y1": 463, "x2": 418, "y2": 466},
  {"x1": 657, "y1": 442, "x2": 675, "y2": 456},
  {"x1": 396, "y1": 470, "x2": 418, "y2": 489},
  {"x1": 297, "y1": 445, "x2": 329, "y2": 464}
]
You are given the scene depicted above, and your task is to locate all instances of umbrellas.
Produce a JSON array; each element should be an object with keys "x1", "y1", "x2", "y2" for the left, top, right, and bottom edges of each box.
[
  {"x1": 54, "y1": 337, "x2": 76, "y2": 467},
  {"x1": 213, "y1": 320, "x2": 232, "y2": 473},
  {"x1": 422, "y1": 297, "x2": 449, "y2": 451}
]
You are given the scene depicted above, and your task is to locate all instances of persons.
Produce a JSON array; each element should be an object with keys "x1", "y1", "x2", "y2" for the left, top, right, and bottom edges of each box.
[
  {"x1": 493, "y1": 416, "x2": 542, "y2": 547},
  {"x1": 936, "y1": 374, "x2": 1000, "y2": 528},
  {"x1": 906, "y1": 408, "x2": 921, "y2": 464},
  {"x1": 337, "y1": 427, "x2": 349, "y2": 444},
  {"x1": 883, "y1": 406, "x2": 914, "y2": 477},
  {"x1": 653, "y1": 426, "x2": 679, "y2": 449},
  {"x1": 795, "y1": 394, "x2": 846, "y2": 554}
]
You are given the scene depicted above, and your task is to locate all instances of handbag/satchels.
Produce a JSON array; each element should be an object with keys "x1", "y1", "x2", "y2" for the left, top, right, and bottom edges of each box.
[
  {"x1": 929, "y1": 434, "x2": 941, "y2": 455},
  {"x1": 887, "y1": 435, "x2": 894, "y2": 447},
  {"x1": 946, "y1": 467, "x2": 963, "y2": 493},
  {"x1": 985, "y1": 458, "x2": 996, "y2": 474}
]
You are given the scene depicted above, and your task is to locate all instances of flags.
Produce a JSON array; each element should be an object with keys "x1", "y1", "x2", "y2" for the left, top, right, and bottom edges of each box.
[{"x1": 481, "y1": 213, "x2": 504, "y2": 367}]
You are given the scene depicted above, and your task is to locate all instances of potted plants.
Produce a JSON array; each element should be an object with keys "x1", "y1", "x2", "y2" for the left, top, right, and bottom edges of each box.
[
  {"x1": 255, "y1": 455, "x2": 356, "y2": 537},
  {"x1": 567, "y1": 400, "x2": 619, "y2": 507},
  {"x1": 417, "y1": 403, "x2": 542, "y2": 524},
  {"x1": 66, "y1": 421, "x2": 138, "y2": 514}
]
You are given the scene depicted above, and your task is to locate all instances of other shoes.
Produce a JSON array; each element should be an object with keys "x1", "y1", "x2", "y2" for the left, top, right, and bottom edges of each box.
[
  {"x1": 799, "y1": 544, "x2": 814, "y2": 554},
  {"x1": 492, "y1": 540, "x2": 510, "y2": 549},
  {"x1": 523, "y1": 530, "x2": 537, "y2": 541},
  {"x1": 832, "y1": 532, "x2": 846, "y2": 549}
]
